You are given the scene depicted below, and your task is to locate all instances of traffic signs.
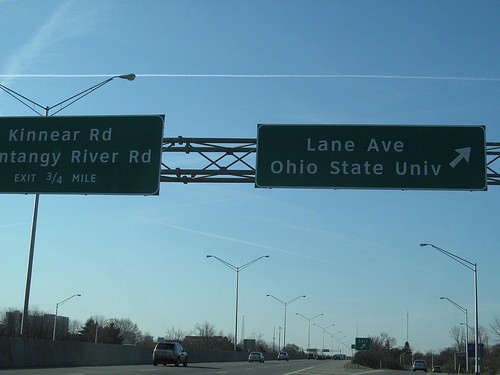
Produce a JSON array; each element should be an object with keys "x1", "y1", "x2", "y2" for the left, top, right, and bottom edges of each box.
[{"x1": 255, "y1": 123, "x2": 488, "y2": 191}]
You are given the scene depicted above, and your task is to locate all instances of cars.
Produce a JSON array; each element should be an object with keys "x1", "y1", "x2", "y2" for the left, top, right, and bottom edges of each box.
[
  {"x1": 248, "y1": 351, "x2": 265, "y2": 363},
  {"x1": 306, "y1": 353, "x2": 352, "y2": 361},
  {"x1": 431, "y1": 366, "x2": 441, "y2": 372},
  {"x1": 411, "y1": 360, "x2": 428, "y2": 372},
  {"x1": 277, "y1": 351, "x2": 290, "y2": 361}
]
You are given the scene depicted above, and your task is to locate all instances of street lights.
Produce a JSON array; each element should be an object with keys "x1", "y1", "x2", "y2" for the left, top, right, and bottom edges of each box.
[
  {"x1": 266, "y1": 294, "x2": 306, "y2": 351},
  {"x1": 0, "y1": 72, "x2": 136, "y2": 336},
  {"x1": 334, "y1": 335, "x2": 346, "y2": 355},
  {"x1": 95, "y1": 318, "x2": 113, "y2": 344},
  {"x1": 313, "y1": 323, "x2": 335, "y2": 355},
  {"x1": 420, "y1": 243, "x2": 478, "y2": 373},
  {"x1": 323, "y1": 330, "x2": 342, "y2": 355},
  {"x1": 440, "y1": 296, "x2": 469, "y2": 372},
  {"x1": 206, "y1": 254, "x2": 269, "y2": 352},
  {"x1": 52, "y1": 293, "x2": 81, "y2": 342},
  {"x1": 296, "y1": 313, "x2": 323, "y2": 358}
]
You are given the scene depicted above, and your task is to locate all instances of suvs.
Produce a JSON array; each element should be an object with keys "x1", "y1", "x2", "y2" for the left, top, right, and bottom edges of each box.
[{"x1": 152, "y1": 341, "x2": 188, "y2": 368}]
